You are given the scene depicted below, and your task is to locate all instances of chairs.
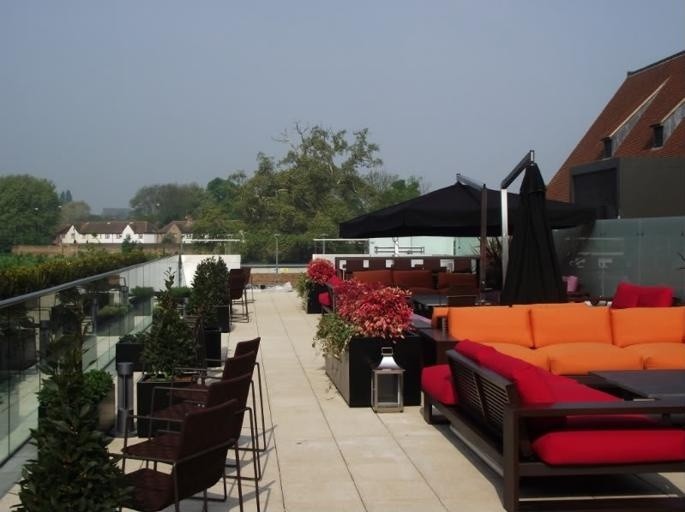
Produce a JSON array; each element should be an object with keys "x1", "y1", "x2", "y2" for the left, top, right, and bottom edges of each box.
[
  {"x1": 445, "y1": 294, "x2": 478, "y2": 308},
  {"x1": 100, "y1": 336, "x2": 272, "y2": 512}
]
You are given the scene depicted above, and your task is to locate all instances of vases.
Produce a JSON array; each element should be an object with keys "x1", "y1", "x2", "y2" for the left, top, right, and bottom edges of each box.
[
  {"x1": 302, "y1": 281, "x2": 329, "y2": 315},
  {"x1": 324, "y1": 331, "x2": 420, "y2": 408}
]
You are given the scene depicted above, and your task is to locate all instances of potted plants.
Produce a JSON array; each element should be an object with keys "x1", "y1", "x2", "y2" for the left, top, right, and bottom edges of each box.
[
  {"x1": 95, "y1": 254, "x2": 232, "y2": 437},
  {"x1": 225, "y1": 266, "x2": 251, "y2": 323}
]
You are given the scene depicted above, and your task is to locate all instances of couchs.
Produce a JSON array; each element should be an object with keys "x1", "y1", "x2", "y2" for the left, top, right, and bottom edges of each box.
[
  {"x1": 347, "y1": 268, "x2": 480, "y2": 306},
  {"x1": 418, "y1": 304, "x2": 683, "y2": 384},
  {"x1": 421, "y1": 338, "x2": 683, "y2": 511}
]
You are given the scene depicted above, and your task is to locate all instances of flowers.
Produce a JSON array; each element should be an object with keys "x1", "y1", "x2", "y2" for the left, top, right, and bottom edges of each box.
[
  {"x1": 307, "y1": 280, "x2": 414, "y2": 352},
  {"x1": 303, "y1": 256, "x2": 337, "y2": 286}
]
